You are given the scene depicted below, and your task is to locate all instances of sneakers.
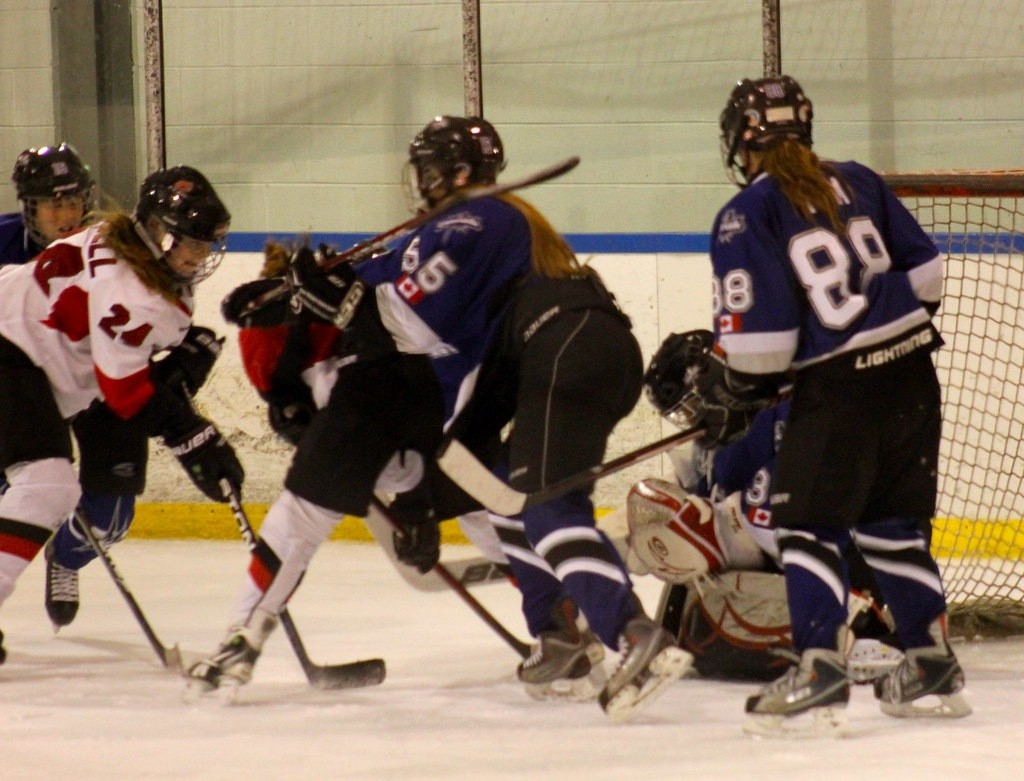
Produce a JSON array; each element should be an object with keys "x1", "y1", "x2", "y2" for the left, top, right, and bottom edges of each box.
[
  {"x1": 184, "y1": 624, "x2": 260, "y2": 704},
  {"x1": 872, "y1": 611, "x2": 972, "y2": 719},
  {"x1": 743, "y1": 624, "x2": 855, "y2": 741},
  {"x1": 598, "y1": 609, "x2": 695, "y2": 724},
  {"x1": 43, "y1": 541, "x2": 80, "y2": 632},
  {"x1": 517, "y1": 607, "x2": 608, "y2": 701}
]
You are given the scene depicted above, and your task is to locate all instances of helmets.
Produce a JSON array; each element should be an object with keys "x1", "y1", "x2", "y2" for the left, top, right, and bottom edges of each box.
[
  {"x1": 401, "y1": 116, "x2": 506, "y2": 214},
  {"x1": 135, "y1": 164, "x2": 231, "y2": 289},
  {"x1": 720, "y1": 74, "x2": 814, "y2": 190},
  {"x1": 644, "y1": 327, "x2": 776, "y2": 454},
  {"x1": 10, "y1": 140, "x2": 96, "y2": 249}
]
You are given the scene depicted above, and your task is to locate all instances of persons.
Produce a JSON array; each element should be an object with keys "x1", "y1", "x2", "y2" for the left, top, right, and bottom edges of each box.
[
  {"x1": 178, "y1": 239, "x2": 607, "y2": 706},
  {"x1": 692, "y1": 76, "x2": 975, "y2": 741},
  {"x1": 289, "y1": 114, "x2": 695, "y2": 721},
  {"x1": 0, "y1": 142, "x2": 136, "y2": 636},
  {"x1": 0, "y1": 164, "x2": 245, "y2": 663},
  {"x1": 628, "y1": 329, "x2": 886, "y2": 680}
]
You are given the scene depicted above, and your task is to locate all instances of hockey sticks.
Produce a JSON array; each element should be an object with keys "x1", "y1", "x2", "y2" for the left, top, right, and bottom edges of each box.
[
  {"x1": 434, "y1": 385, "x2": 794, "y2": 519},
  {"x1": 73, "y1": 510, "x2": 210, "y2": 671},
  {"x1": 236, "y1": 154, "x2": 582, "y2": 318},
  {"x1": 183, "y1": 378, "x2": 386, "y2": 692},
  {"x1": 366, "y1": 498, "x2": 628, "y2": 592},
  {"x1": 369, "y1": 490, "x2": 606, "y2": 669}
]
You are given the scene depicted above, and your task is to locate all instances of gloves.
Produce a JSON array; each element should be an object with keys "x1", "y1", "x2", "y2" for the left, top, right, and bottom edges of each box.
[
  {"x1": 219, "y1": 273, "x2": 311, "y2": 328},
  {"x1": 164, "y1": 417, "x2": 245, "y2": 504},
  {"x1": 388, "y1": 482, "x2": 442, "y2": 574},
  {"x1": 690, "y1": 368, "x2": 779, "y2": 451},
  {"x1": 287, "y1": 243, "x2": 366, "y2": 332},
  {"x1": 168, "y1": 323, "x2": 224, "y2": 398}
]
